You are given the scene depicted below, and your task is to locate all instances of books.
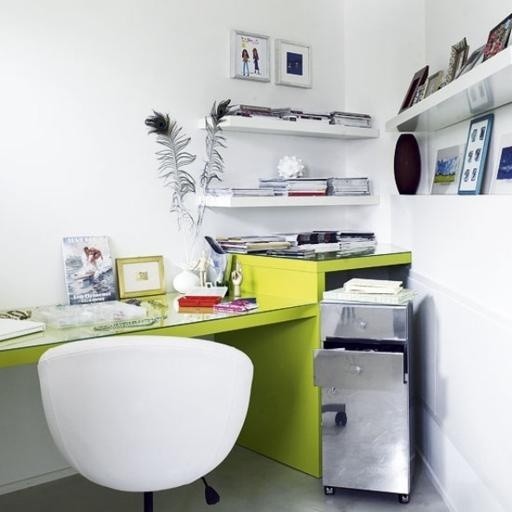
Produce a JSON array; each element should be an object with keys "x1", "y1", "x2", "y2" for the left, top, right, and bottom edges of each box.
[
  {"x1": 62, "y1": 235, "x2": 120, "y2": 304},
  {"x1": 205, "y1": 101, "x2": 380, "y2": 260}
]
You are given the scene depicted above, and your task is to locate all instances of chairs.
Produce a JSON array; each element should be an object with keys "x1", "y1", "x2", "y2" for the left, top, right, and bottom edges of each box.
[{"x1": 35, "y1": 334, "x2": 255, "y2": 512}]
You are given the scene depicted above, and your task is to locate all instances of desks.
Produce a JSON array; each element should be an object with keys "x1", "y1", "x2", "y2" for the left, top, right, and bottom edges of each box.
[
  {"x1": 0, "y1": 289, "x2": 320, "y2": 482},
  {"x1": 224, "y1": 249, "x2": 413, "y2": 478}
]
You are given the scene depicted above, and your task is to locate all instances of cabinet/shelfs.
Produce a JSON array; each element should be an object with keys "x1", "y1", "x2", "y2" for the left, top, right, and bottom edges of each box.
[
  {"x1": 198, "y1": 115, "x2": 379, "y2": 207},
  {"x1": 310, "y1": 290, "x2": 418, "y2": 504},
  {"x1": 385, "y1": 44, "x2": 511, "y2": 205}
]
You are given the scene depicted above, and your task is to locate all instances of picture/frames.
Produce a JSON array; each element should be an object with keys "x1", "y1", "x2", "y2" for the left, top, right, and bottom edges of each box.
[
  {"x1": 115, "y1": 256, "x2": 167, "y2": 299},
  {"x1": 229, "y1": 28, "x2": 313, "y2": 90},
  {"x1": 457, "y1": 113, "x2": 495, "y2": 195},
  {"x1": 397, "y1": 12, "x2": 512, "y2": 114}
]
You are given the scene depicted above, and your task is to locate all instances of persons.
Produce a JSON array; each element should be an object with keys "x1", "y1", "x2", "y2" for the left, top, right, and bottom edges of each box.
[{"x1": 84, "y1": 246, "x2": 102, "y2": 270}]
